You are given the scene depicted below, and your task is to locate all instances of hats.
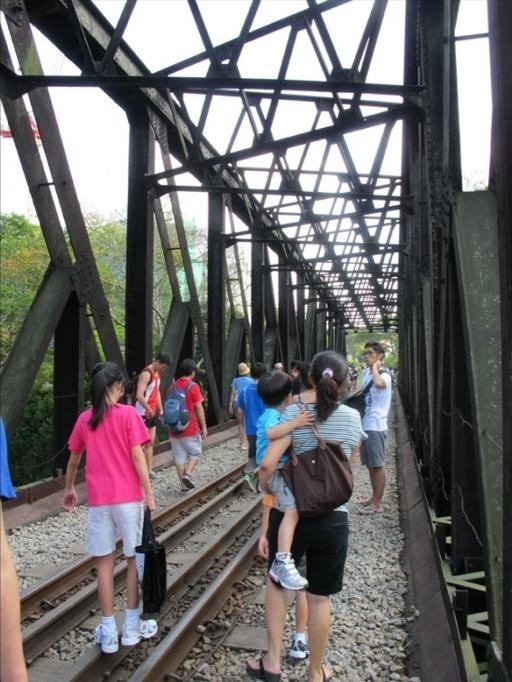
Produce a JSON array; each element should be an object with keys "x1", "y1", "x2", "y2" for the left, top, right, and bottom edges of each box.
[{"x1": 238, "y1": 363, "x2": 250, "y2": 375}]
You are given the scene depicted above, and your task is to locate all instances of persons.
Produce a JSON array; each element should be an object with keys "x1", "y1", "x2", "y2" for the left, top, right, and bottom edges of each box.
[
  {"x1": 0, "y1": 417, "x2": 29, "y2": 682},
  {"x1": 132, "y1": 353, "x2": 163, "y2": 481},
  {"x1": 62, "y1": 359, "x2": 159, "y2": 653},
  {"x1": 164, "y1": 357, "x2": 208, "y2": 495},
  {"x1": 228, "y1": 340, "x2": 399, "y2": 681}
]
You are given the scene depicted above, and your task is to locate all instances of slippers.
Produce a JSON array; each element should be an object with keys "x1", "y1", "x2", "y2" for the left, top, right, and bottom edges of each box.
[
  {"x1": 307, "y1": 665, "x2": 331, "y2": 682},
  {"x1": 247, "y1": 656, "x2": 280, "y2": 682}
]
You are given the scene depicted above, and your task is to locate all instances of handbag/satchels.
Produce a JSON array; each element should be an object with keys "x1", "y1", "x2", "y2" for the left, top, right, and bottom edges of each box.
[
  {"x1": 344, "y1": 395, "x2": 366, "y2": 418},
  {"x1": 280, "y1": 441, "x2": 353, "y2": 517},
  {"x1": 135, "y1": 542, "x2": 166, "y2": 615}
]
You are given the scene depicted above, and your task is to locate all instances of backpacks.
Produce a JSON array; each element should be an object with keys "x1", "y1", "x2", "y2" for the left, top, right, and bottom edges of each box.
[
  {"x1": 164, "y1": 380, "x2": 195, "y2": 434},
  {"x1": 120, "y1": 369, "x2": 153, "y2": 405}
]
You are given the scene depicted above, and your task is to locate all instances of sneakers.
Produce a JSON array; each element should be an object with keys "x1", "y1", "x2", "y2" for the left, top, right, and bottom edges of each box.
[
  {"x1": 121, "y1": 618, "x2": 158, "y2": 646},
  {"x1": 94, "y1": 624, "x2": 119, "y2": 653},
  {"x1": 288, "y1": 638, "x2": 310, "y2": 659},
  {"x1": 243, "y1": 474, "x2": 255, "y2": 491},
  {"x1": 269, "y1": 552, "x2": 309, "y2": 590},
  {"x1": 180, "y1": 474, "x2": 195, "y2": 494}
]
